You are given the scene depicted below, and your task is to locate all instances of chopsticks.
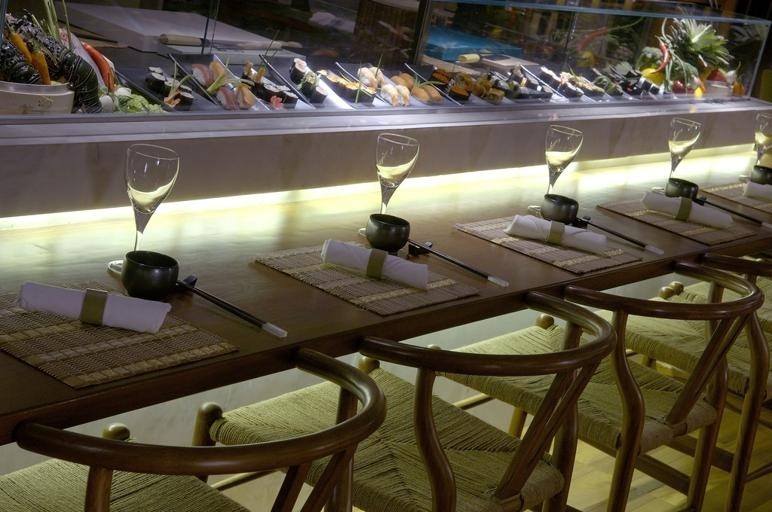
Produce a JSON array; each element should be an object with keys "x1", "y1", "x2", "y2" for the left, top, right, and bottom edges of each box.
[
  {"x1": 405, "y1": 237, "x2": 510, "y2": 289},
  {"x1": 175, "y1": 276, "x2": 288, "y2": 341},
  {"x1": 574, "y1": 215, "x2": 665, "y2": 255},
  {"x1": 693, "y1": 196, "x2": 772, "y2": 229}
]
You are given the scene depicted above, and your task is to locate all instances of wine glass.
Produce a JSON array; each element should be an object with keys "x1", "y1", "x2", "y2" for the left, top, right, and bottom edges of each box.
[
  {"x1": 105, "y1": 142, "x2": 183, "y2": 275},
  {"x1": 528, "y1": 124, "x2": 584, "y2": 218},
  {"x1": 738, "y1": 112, "x2": 772, "y2": 184},
  {"x1": 358, "y1": 133, "x2": 420, "y2": 238},
  {"x1": 652, "y1": 117, "x2": 703, "y2": 194}
]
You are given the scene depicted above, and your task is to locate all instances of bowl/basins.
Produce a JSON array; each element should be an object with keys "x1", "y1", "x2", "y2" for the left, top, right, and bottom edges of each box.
[
  {"x1": 750, "y1": 165, "x2": 772, "y2": 185},
  {"x1": 541, "y1": 194, "x2": 579, "y2": 225},
  {"x1": 120, "y1": 249, "x2": 180, "y2": 299},
  {"x1": 666, "y1": 178, "x2": 698, "y2": 202},
  {"x1": 365, "y1": 212, "x2": 411, "y2": 254}
]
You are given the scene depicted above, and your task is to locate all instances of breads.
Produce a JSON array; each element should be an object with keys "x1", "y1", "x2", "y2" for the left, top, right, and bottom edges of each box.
[{"x1": 430, "y1": 68, "x2": 504, "y2": 105}]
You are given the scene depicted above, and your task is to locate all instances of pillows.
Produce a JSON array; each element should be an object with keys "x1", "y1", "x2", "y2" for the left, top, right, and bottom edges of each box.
[
  {"x1": 17, "y1": 281, "x2": 171, "y2": 333},
  {"x1": 504, "y1": 215, "x2": 606, "y2": 256},
  {"x1": 642, "y1": 189, "x2": 732, "y2": 229},
  {"x1": 744, "y1": 182, "x2": 772, "y2": 199},
  {"x1": 321, "y1": 239, "x2": 429, "y2": 290}
]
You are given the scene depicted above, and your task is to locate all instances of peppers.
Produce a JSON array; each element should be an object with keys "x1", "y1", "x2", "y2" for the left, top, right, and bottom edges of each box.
[
  {"x1": 31, "y1": 40, "x2": 51, "y2": 85},
  {"x1": 6, "y1": 21, "x2": 31, "y2": 64},
  {"x1": 80, "y1": 40, "x2": 116, "y2": 93},
  {"x1": 650, "y1": 34, "x2": 669, "y2": 73}
]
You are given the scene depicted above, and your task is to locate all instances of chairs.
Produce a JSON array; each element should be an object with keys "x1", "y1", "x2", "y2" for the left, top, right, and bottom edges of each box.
[
  {"x1": 0, "y1": 346, "x2": 386, "y2": 511},
  {"x1": 670, "y1": 255, "x2": 768, "y2": 334},
  {"x1": 191, "y1": 291, "x2": 617, "y2": 512},
  {"x1": 566, "y1": 262, "x2": 772, "y2": 512}
]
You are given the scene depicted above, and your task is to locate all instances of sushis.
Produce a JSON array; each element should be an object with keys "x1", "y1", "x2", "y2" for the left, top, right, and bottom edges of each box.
[
  {"x1": 509, "y1": 66, "x2": 585, "y2": 100},
  {"x1": 607, "y1": 69, "x2": 660, "y2": 95},
  {"x1": 243, "y1": 57, "x2": 374, "y2": 108},
  {"x1": 147, "y1": 65, "x2": 194, "y2": 108}
]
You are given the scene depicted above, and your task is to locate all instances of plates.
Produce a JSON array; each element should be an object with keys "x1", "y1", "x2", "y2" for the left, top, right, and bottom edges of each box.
[
  {"x1": 168, "y1": 52, "x2": 270, "y2": 110},
  {"x1": 259, "y1": 53, "x2": 355, "y2": 111},
  {"x1": 214, "y1": 53, "x2": 317, "y2": 111},
  {"x1": 310, "y1": 53, "x2": 678, "y2": 111},
  {"x1": 115, "y1": 52, "x2": 224, "y2": 111}
]
